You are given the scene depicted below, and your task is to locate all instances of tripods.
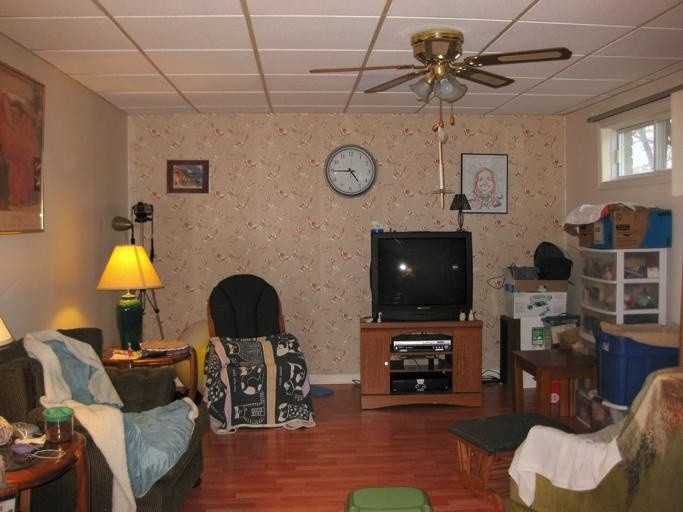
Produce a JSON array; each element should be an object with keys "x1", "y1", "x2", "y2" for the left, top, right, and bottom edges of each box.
[{"x1": 133, "y1": 224, "x2": 166, "y2": 341}]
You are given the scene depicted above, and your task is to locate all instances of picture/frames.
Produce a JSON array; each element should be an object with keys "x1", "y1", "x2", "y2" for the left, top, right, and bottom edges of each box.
[
  {"x1": 0, "y1": 61, "x2": 46, "y2": 234},
  {"x1": 165, "y1": 159, "x2": 209, "y2": 193},
  {"x1": 459, "y1": 153, "x2": 509, "y2": 215}
]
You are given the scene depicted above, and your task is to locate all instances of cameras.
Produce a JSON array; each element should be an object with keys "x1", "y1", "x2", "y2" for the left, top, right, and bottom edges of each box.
[{"x1": 132, "y1": 202, "x2": 153, "y2": 223}]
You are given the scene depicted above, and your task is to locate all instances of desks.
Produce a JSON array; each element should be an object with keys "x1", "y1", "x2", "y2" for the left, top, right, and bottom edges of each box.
[{"x1": 514, "y1": 350, "x2": 596, "y2": 423}]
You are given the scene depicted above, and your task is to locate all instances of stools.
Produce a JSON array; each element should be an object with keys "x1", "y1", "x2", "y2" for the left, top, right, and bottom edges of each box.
[{"x1": 348, "y1": 486, "x2": 432, "y2": 511}]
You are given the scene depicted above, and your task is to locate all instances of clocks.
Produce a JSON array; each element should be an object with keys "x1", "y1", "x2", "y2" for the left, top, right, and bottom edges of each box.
[{"x1": 325, "y1": 146, "x2": 379, "y2": 195}]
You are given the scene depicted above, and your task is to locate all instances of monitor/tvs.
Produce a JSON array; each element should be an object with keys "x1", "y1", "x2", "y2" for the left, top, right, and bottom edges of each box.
[{"x1": 371, "y1": 231, "x2": 474, "y2": 323}]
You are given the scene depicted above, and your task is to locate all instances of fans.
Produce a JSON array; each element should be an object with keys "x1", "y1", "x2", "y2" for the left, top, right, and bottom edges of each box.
[{"x1": 308, "y1": 29, "x2": 572, "y2": 132}]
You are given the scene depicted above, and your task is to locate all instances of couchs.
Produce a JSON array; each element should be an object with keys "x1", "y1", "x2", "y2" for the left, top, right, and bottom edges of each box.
[
  {"x1": 1, "y1": 328, "x2": 213, "y2": 511},
  {"x1": 202, "y1": 273, "x2": 317, "y2": 436},
  {"x1": 507, "y1": 369, "x2": 683, "y2": 511}
]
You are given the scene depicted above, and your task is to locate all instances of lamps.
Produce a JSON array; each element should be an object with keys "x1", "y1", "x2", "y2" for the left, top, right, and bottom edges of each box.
[
  {"x1": 449, "y1": 194, "x2": 471, "y2": 231},
  {"x1": 96, "y1": 243, "x2": 166, "y2": 351}
]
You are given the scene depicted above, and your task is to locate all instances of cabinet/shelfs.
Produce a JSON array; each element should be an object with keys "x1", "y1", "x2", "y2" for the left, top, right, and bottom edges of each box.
[
  {"x1": 577, "y1": 250, "x2": 667, "y2": 431},
  {"x1": 359, "y1": 320, "x2": 484, "y2": 408}
]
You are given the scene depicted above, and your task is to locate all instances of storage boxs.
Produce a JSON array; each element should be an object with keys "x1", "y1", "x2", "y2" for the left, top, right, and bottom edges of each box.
[
  {"x1": 591, "y1": 322, "x2": 680, "y2": 407},
  {"x1": 502, "y1": 266, "x2": 574, "y2": 387},
  {"x1": 561, "y1": 201, "x2": 672, "y2": 248}
]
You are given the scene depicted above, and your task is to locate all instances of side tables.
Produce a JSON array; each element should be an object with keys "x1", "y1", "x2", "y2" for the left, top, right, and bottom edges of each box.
[
  {"x1": 102, "y1": 344, "x2": 199, "y2": 402},
  {"x1": 0, "y1": 429, "x2": 89, "y2": 512}
]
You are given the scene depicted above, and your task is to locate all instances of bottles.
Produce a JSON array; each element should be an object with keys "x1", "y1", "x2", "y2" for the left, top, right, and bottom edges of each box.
[{"x1": 43, "y1": 408, "x2": 73, "y2": 444}]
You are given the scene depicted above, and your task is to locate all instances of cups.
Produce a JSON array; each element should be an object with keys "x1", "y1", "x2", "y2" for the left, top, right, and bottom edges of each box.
[{"x1": 42, "y1": 404, "x2": 76, "y2": 445}]
